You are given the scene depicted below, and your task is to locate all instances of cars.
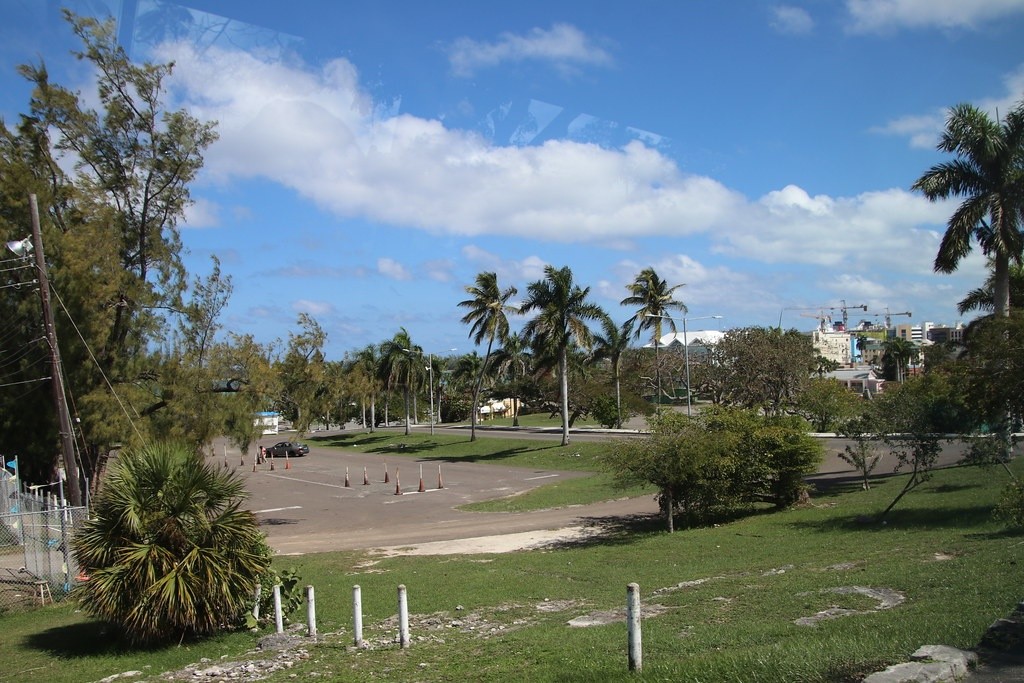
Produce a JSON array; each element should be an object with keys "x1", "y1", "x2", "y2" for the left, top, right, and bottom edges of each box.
[{"x1": 266, "y1": 442, "x2": 309, "y2": 458}]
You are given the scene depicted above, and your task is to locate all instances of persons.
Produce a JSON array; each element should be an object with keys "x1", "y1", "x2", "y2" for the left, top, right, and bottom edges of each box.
[{"x1": 258, "y1": 446, "x2": 268, "y2": 464}]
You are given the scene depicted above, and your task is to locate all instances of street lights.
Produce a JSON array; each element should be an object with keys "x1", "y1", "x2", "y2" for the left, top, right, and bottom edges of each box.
[
  {"x1": 644, "y1": 314, "x2": 723, "y2": 420},
  {"x1": 403, "y1": 349, "x2": 457, "y2": 426}
]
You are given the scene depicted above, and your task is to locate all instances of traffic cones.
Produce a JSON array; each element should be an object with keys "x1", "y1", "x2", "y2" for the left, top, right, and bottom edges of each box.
[
  {"x1": 345, "y1": 467, "x2": 350, "y2": 487},
  {"x1": 224, "y1": 444, "x2": 229, "y2": 467},
  {"x1": 363, "y1": 466, "x2": 371, "y2": 485},
  {"x1": 418, "y1": 464, "x2": 425, "y2": 492},
  {"x1": 384, "y1": 464, "x2": 391, "y2": 483},
  {"x1": 286, "y1": 451, "x2": 290, "y2": 469},
  {"x1": 394, "y1": 467, "x2": 403, "y2": 495},
  {"x1": 437, "y1": 465, "x2": 444, "y2": 488},
  {"x1": 252, "y1": 453, "x2": 258, "y2": 472},
  {"x1": 240, "y1": 454, "x2": 245, "y2": 465},
  {"x1": 271, "y1": 453, "x2": 275, "y2": 470}
]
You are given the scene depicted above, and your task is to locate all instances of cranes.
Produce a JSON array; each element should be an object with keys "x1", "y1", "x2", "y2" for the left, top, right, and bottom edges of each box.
[
  {"x1": 847, "y1": 308, "x2": 913, "y2": 328},
  {"x1": 785, "y1": 299, "x2": 867, "y2": 331}
]
